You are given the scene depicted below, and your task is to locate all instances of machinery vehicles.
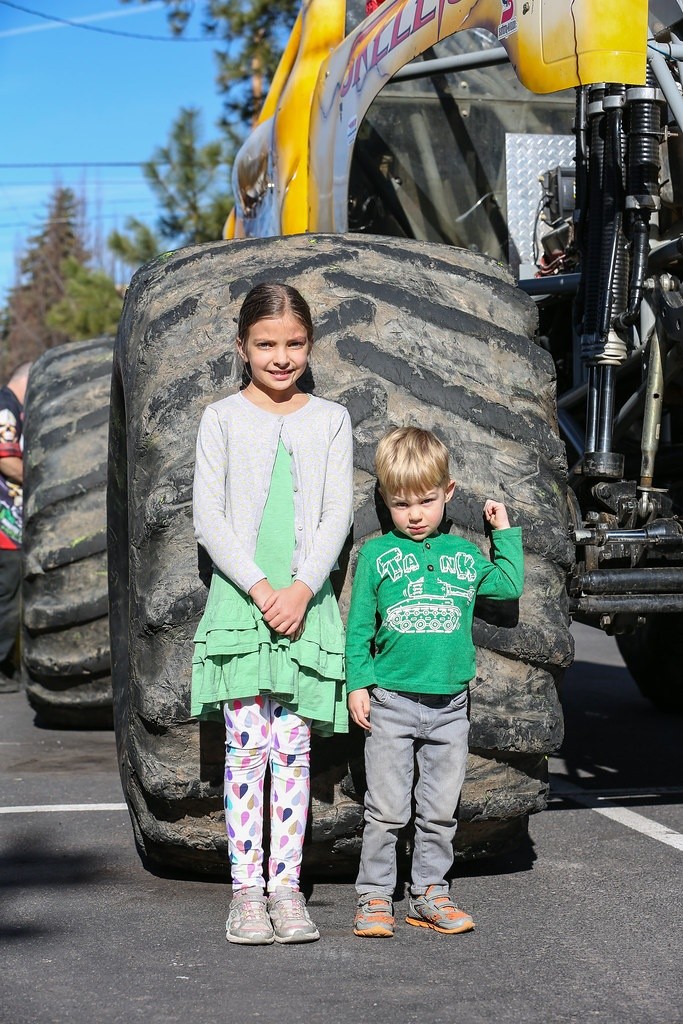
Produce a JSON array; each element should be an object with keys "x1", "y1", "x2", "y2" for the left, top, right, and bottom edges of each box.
[{"x1": 0, "y1": 0, "x2": 683, "y2": 883}]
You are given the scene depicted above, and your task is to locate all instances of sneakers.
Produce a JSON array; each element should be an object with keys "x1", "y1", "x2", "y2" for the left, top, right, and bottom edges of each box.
[
  {"x1": 268, "y1": 885, "x2": 320, "y2": 943},
  {"x1": 353, "y1": 892, "x2": 396, "y2": 936},
  {"x1": 406, "y1": 885, "x2": 474, "y2": 933},
  {"x1": 226, "y1": 887, "x2": 274, "y2": 943}
]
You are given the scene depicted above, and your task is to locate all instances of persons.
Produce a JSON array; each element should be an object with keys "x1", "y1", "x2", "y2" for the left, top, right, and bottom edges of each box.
[
  {"x1": 0, "y1": 361, "x2": 28, "y2": 693},
  {"x1": 346, "y1": 427, "x2": 524, "y2": 939},
  {"x1": 190, "y1": 283, "x2": 355, "y2": 943}
]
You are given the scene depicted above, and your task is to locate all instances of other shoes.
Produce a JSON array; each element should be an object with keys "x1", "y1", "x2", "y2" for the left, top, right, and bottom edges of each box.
[{"x1": 0, "y1": 672, "x2": 21, "y2": 692}]
